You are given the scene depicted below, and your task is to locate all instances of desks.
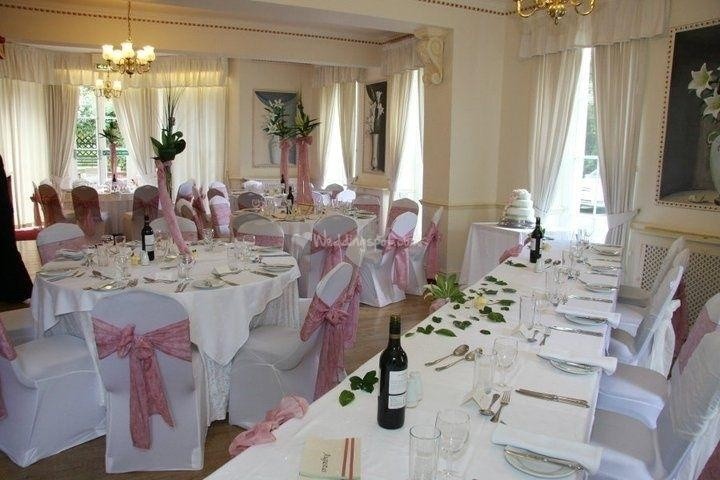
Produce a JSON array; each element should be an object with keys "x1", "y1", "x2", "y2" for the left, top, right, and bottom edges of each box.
[{"x1": 459, "y1": 221, "x2": 533, "y2": 288}]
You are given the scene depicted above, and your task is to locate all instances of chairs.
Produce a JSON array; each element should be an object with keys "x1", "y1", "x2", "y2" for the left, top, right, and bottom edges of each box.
[{"x1": 588, "y1": 236, "x2": 719, "y2": 480}]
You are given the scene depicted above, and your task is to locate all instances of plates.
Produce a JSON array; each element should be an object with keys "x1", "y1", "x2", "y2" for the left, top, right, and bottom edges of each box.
[
  {"x1": 504, "y1": 445, "x2": 583, "y2": 478},
  {"x1": 550, "y1": 359, "x2": 597, "y2": 375},
  {"x1": 37, "y1": 243, "x2": 295, "y2": 292},
  {"x1": 565, "y1": 314, "x2": 606, "y2": 326},
  {"x1": 585, "y1": 285, "x2": 615, "y2": 293}
]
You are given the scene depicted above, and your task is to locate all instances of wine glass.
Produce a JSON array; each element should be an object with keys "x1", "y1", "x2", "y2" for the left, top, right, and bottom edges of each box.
[
  {"x1": 433, "y1": 408, "x2": 473, "y2": 480},
  {"x1": 490, "y1": 335, "x2": 519, "y2": 392}
]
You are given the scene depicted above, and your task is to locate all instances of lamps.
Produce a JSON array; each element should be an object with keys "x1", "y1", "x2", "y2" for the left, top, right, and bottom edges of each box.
[
  {"x1": 102, "y1": 0, "x2": 157, "y2": 77},
  {"x1": 91, "y1": 71, "x2": 122, "y2": 99},
  {"x1": 516, "y1": 2, "x2": 597, "y2": 22}
]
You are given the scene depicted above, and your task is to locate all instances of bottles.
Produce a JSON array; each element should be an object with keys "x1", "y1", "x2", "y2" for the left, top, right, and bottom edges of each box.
[
  {"x1": 281, "y1": 175, "x2": 286, "y2": 193},
  {"x1": 142, "y1": 216, "x2": 155, "y2": 261},
  {"x1": 376, "y1": 311, "x2": 409, "y2": 431}
]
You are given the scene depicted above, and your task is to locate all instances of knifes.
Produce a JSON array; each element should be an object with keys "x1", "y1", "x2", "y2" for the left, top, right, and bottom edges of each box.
[
  {"x1": 536, "y1": 354, "x2": 592, "y2": 372},
  {"x1": 515, "y1": 388, "x2": 590, "y2": 408},
  {"x1": 503, "y1": 449, "x2": 583, "y2": 471}
]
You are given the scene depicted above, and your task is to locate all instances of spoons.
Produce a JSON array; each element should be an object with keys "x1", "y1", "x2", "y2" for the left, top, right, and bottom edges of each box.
[
  {"x1": 425, "y1": 344, "x2": 469, "y2": 367},
  {"x1": 435, "y1": 347, "x2": 483, "y2": 372},
  {"x1": 478, "y1": 393, "x2": 500, "y2": 417}
]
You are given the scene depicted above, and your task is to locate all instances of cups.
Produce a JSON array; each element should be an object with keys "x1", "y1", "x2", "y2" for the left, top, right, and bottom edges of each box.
[
  {"x1": 407, "y1": 422, "x2": 442, "y2": 480},
  {"x1": 404, "y1": 369, "x2": 424, "y2": 409},
  {"x1": 94, "y1": 228, "x2": 256, "y2": 283},
  {"x1": 516, "y1": 217, "x2": 595, "y2": 331},
  {"x1": 471, "y1": 348, "x2": 498, "y2": 396},
  {"x1": 72, "y1": 179, "x2": 138, "y2": 195},
  {"x1": 260, "y1": 182, "x2": 356, "y2": 217}
]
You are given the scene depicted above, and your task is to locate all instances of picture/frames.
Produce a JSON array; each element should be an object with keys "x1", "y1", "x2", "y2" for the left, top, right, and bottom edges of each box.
[
  {"x1": 653, "y1": 19, "x2": 719, "y2": 209},
  {"x1": 361, "y1": 79, "x2": 389, "y2": 174},
  {"x1": 251, "y1": 89, "x2": 299, "y2": 169}
]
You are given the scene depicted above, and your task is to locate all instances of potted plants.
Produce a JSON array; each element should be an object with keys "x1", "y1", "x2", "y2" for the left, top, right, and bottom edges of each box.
[
  {"x1": 266, "y1": 99, "x2": 293, "y2": 205},
  {"x1": 284, "y1": 98, "x2": 321, "y2": 202},
  {"x1": 149, "y1": 81, "x2": 188, "y2": 199},
  {"x1": 99, "y1": 118, "x2": 122, "y2": 173},
  {"x1": 421, "y1": 270, "x2": 462, "y2": 315}
]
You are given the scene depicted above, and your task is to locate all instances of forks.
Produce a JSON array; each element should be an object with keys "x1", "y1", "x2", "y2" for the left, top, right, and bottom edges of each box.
[{"x1": 490, "y1": 391, "x2": 512, "y2": 423}]
